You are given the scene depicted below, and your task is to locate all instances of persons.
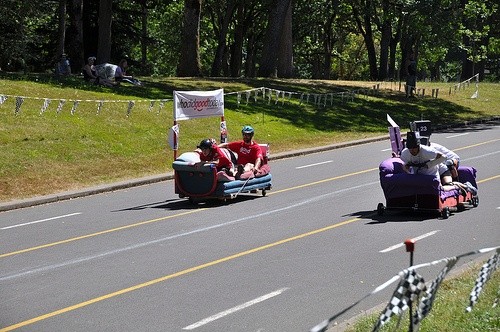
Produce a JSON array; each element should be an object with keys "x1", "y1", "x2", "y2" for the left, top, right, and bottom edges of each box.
[
  {"x1": 83, "y1": 56, "x2": 120, "y2": 88},
  {"x1": 195, "y1": 138, "x2": 234, "y2": 180},
  {"x1": 400, "y1": 137, "x2": 477, "y2": 197},
  {"x1": 54, "y1": 53, "x2": 78, "y2": 78},
  {"x1": 217, "y1": 125, "x2": 264, "y2": 175},
  {"x1": 115, "y1": 59, "x2": 141, "y2": 85}
]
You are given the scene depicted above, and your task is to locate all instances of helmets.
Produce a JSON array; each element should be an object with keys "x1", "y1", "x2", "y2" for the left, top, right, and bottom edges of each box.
[
  {"x1": 200, "y1": 139, "x2": 211, "y2": 147},
  {"x1": 242, "y1": 126, "x2": 254, "y2": 134}
]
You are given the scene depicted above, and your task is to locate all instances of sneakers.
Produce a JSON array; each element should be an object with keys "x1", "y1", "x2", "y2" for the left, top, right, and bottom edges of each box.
[{"x1": 465, "y1": 182, "x2": 477, "y2": 195}]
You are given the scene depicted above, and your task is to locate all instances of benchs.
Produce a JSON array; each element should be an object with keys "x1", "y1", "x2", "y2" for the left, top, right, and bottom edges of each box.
[
  {"x1": 172, "y1": 145, "x2": 272, "y2": 195},
  {"x1": 380, "y1": 153, "x2": 478, "y2": 210}
]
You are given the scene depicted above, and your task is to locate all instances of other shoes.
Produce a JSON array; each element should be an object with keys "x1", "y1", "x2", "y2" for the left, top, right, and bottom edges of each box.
[{"x1": 235, "y1": 164, "x2": 243, "y2": 179}]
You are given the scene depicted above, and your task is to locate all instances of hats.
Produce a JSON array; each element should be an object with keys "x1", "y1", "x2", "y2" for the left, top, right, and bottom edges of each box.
[{"x1": 406, "y1": 131, "x2": 420, "y2": 145}]
